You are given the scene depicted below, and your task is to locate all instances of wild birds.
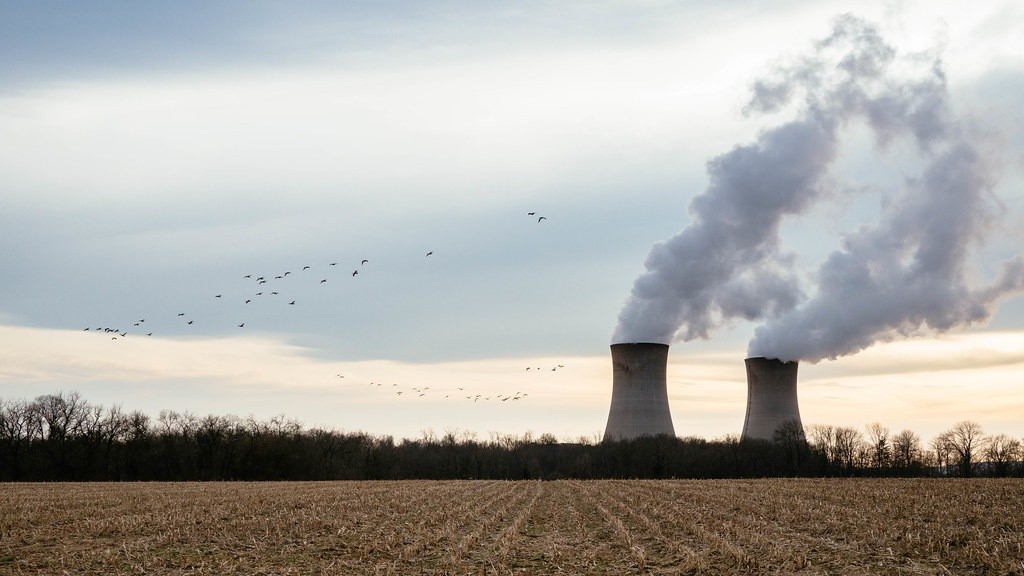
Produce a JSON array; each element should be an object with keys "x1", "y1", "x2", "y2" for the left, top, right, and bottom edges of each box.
[
  {"x1": 84, "y1": 327, "x2": 90, "y2": 332},
  {"x1": 133, "y1": 318, "x2": 145, "y2": 326},
  {"x1": 146, "y1": 332, "x2": 153, "y2": 336},
  {"x1": 97, "y1": 327, "x2": 127, "y2": 340},
  {"x1": 352, "y1": 259, "x2": 369, "y2": 277},
  {"x1": 215, "y1": 295, "x2": 221, "y2": 298},
  {"x1": 528, "y1": 212, "x2": 547, "y2": 223},
  {"x1": 238, "y1": 261, "x2": 338, "y2": 328},
  {"x1": 337, "y1": 363, "x2": 566, "y2": 404},
  {"x1": 425, "y1": 250, "x2": 434, "y2": 258},
  {"x1": 178, "y1": 313, "x2": 184, "y2": 317},
  {"x1": 187, "y1": 320, "x2": 194, "y2": 325}
]
